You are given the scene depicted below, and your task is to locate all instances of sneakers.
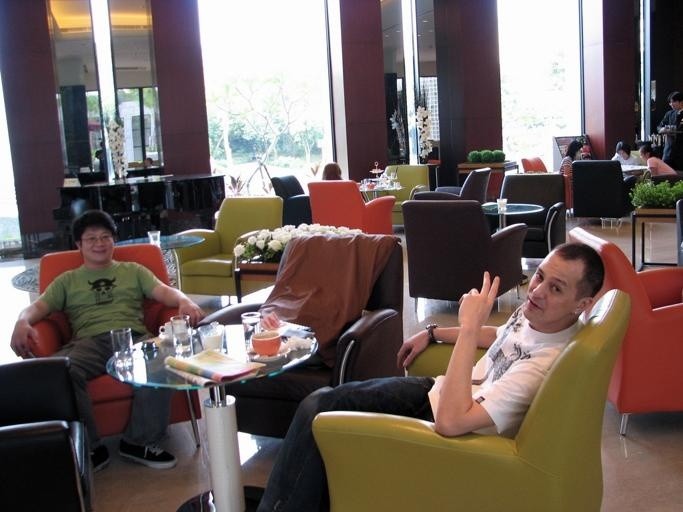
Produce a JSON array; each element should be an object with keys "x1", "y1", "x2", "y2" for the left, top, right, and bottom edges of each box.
[{"x1": 90, "y1": 439, "x2": 177, "y2": 473}]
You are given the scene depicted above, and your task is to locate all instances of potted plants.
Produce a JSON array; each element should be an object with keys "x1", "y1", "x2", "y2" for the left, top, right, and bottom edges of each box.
[{"x1": 466, "y1": 149, "x2": 506, "y2": 164}]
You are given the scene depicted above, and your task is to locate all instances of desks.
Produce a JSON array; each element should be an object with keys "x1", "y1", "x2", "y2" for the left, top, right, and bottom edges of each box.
[{"x1": 105, "y1": 320, "x2": 320, "y2": 512}]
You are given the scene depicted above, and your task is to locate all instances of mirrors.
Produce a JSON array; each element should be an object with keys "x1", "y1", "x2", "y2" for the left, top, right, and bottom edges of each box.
[
  {"x1": 380, "y1": 1, "x2": 410, "y2": 165},
  {"x1": 106, "y1": 0, "x2": 165, "y2": 177},
  {"x1": 410, "y1": 1, "x2": 440, "y2": 165},
  {"x1": 44, "y1": 0, "x2": 109, "y2": 180}
]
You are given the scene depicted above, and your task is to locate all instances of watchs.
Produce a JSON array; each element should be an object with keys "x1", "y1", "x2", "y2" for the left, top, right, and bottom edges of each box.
[{"x1": 425, "y1": 323, "x2": 438, "y2": 345}]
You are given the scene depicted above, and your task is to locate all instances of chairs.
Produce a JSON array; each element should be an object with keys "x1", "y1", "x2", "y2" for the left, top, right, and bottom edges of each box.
[
  {"x1": 50, "y1": 181, "x2": 170, "y2": 242},
  {"x1": 0, "y1": 356, "x2": 93, "y2": 509},
  {"x1": 310, "y1": 288, "x2": 631, "y2": 511},
  {"x1": 19, "y1": 244, "x2": 205, "y2": 450}
]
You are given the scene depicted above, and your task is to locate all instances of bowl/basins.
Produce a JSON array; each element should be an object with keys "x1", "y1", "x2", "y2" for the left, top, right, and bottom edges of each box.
[{"x1": 251, "y1": 332, "x2": 281, "y2": 356}]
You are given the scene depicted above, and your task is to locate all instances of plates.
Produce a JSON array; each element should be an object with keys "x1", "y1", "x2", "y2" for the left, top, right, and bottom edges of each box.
[{"x1": 158, "y1": 330, "x2": 197, "y2": 338}]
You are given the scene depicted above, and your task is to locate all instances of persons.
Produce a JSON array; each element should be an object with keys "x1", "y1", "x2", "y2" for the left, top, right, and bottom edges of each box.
[
  {"x1": 322, "y1": 162, "x2": 343, "y2": 181},
  {"x1": 9, "y1": 210, "x2": 207, "y2": 471},
  {"x1": 95, "y1": 141, "x2": 154, "y2": 172},
  {"x1": 559, "y1": 141, "x2": 583, "y2": 184},
  {"x1": 610, "y1": 141, "x2": 642, "y2": 177},
  {"x1": 639, "y1": 145, "x2": 678, "y2": 178},
  {"x1": 656, "y1": 91, "x2": 683, "y2": 171},
  {"x1": 257, "y1": 241, "x2": 606, "y2": 511}
]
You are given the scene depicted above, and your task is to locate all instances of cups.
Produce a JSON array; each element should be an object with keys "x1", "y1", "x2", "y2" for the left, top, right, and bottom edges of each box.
[
  {"x1": 159, "y1": 322, "x2": 172, "y2": 335},
  {"x1": 198, "y1": 324, "x2": 224, "y2": 353},
  {"x1": 671, "y1": 125, "x2": 677, "y2": 131},
  {"x1": 361, "y1": 179, "x2": 400, "y2": 189},
  {"x1": 110, "y1": 327, "x2": 133, "y2": 373},
  {"x1": 665, "y1": 125, "x2": 671, "y2": 130},
  {"x1": 148, "y1": 231, "x2": 161, "y2": 247},
  {"x1": 497, "y1": 198, "x2": 508, "y2": 213},
  {"x1": 241, "y1": 312, "x2": 261, "y2": 349}
]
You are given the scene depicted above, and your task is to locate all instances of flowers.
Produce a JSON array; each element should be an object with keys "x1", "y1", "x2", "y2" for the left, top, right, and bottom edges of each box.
[
  {"x1": 105, "y1": 118, "x2": 129, "y2": 179},
  {"x1": 415, "y1": 86, "x2": 433, "y2": 157},
  {"x1": 389, "y1": 108, "x2": 407, "y2": 158}
]
